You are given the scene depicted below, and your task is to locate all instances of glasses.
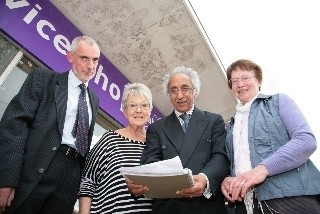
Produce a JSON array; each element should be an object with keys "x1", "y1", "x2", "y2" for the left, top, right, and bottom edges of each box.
[
  {"x1": 124, "y1": 104, "x2": 151, "y2": 110},
  {"x1": 228, "y1": 75, "x2": 256, "y2": 85},
  {"x1": 169, "y1": 87, "x2": 195, "y2": 94}
]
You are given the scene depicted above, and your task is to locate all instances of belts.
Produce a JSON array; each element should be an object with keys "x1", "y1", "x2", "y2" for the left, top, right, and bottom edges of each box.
[{"x1": 59, "y1": 144, "x2": 85, "y2": 163}]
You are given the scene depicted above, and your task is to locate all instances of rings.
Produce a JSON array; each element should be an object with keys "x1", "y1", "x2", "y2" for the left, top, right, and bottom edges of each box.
[{"x1": 188, "y1": 196, "x2": 193, "y2": 198}]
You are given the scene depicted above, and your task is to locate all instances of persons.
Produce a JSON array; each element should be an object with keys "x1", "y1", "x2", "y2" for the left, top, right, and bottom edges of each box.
[
  {"x1": 0, "y1": 36, "x2": 99, "y2": 214},
  {"x1": 126, "y1": 66, "x2": 228, "y2": 214},
  {"x1": 77, "y1": 83, "x2": 153, "y2": 214},
  {"x1": 220, "y1": 58, "x2": 320, "y2": 214}
]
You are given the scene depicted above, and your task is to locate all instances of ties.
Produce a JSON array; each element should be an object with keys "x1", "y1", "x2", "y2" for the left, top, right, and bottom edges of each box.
[
  {"x1": 71, "y1": 83, "x2": 89, "y2": 157},
  {"x1": 179, "y1": 112, "x2": 191, "y2": 134}
]
